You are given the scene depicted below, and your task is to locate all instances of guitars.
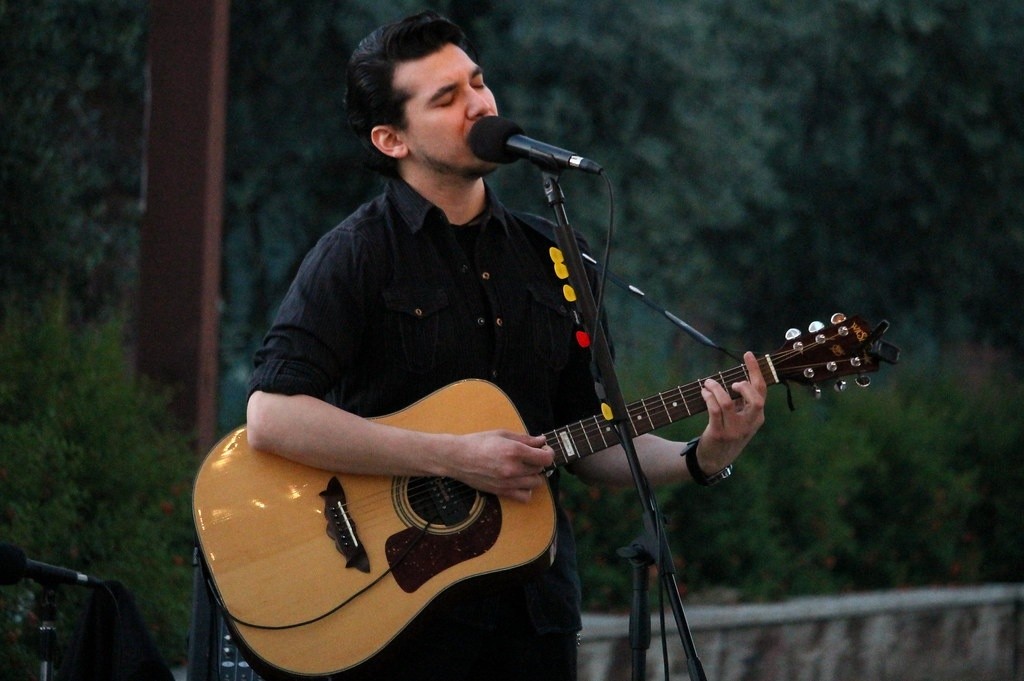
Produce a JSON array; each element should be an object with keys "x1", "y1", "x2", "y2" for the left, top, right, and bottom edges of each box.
[{"x1": 190, "y1": 310, "x2": 901, "y2": 681}]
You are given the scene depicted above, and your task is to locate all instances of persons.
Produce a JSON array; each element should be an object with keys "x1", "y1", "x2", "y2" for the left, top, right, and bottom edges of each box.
[{"x1": 246, "y1": 8, "x2": 766, "y2": 680}]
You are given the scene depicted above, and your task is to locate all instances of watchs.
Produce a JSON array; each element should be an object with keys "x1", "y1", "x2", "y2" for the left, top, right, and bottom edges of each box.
[{"x1": 680, "y1": 434, "x2": 735, "y2": 488}]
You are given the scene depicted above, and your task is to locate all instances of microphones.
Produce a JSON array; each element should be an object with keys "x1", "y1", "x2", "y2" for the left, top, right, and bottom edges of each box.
[
  {"x1": 467, "y1": 115, "x2": 602, "y2": 177},
  {"x1": 0, "y1": 544, "x2": 99, "y2": 590}
]
